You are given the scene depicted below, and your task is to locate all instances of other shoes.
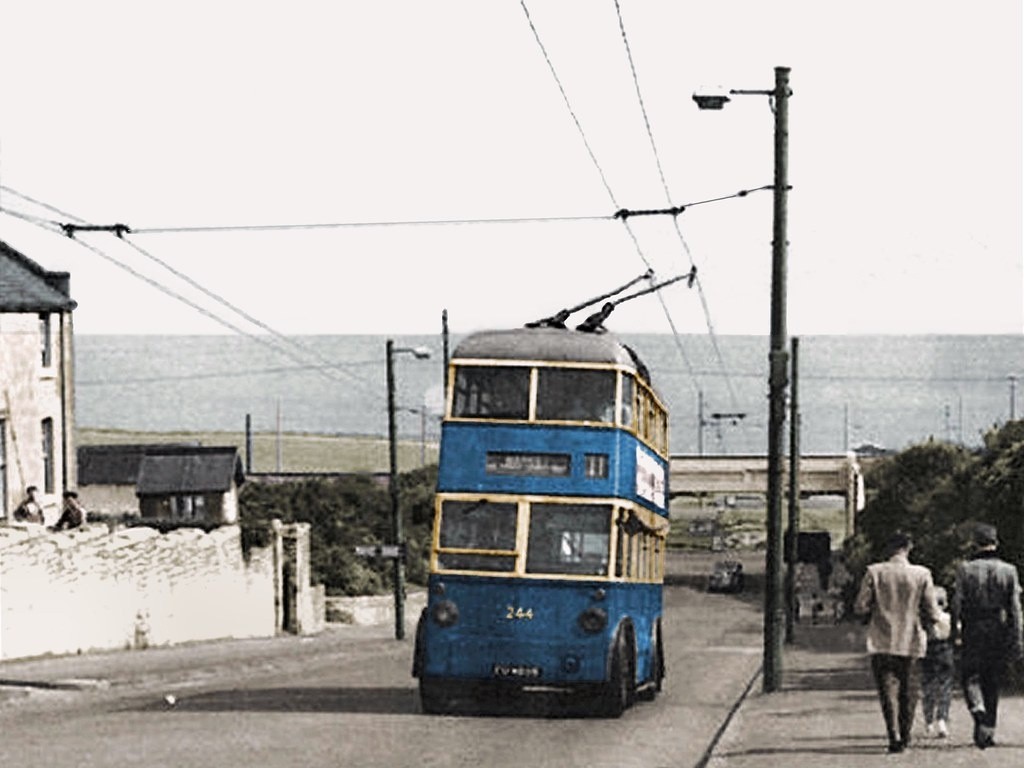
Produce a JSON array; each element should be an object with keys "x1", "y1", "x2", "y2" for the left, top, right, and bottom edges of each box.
[
  {"x1": 889, "y1": 741, "x2": 904, "y2": 753},
  {"x1": 972, "y1": 710, "x2": 988, "y2": 748},
  {"x1": 902, "y1": 733, "x2": 912, "y2": 747},
  {"x1": 937, "y1": 719, "x2": 947, "y2": 737},
  {"x1": 985, "y1": 735, "x2": 994, "y2": 746},
  {"x1": 925, "y1": 724, "x2": 935, "y2": 734}
]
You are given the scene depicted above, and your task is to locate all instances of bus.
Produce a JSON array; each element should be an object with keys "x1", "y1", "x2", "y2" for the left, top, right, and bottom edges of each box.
[{"x1": 411, "y1": 264, "x2": 697, "y2": 719}]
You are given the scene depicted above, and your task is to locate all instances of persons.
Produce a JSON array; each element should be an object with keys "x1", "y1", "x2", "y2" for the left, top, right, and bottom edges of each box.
[
  {"x1": 854, "y1": 525, "x2": 1024, "y2": 754},
  {"x1": 55, "y1": 491, "x2": 86, "y2": 529},
  {"x1": 811, "y1": 592, "x2": 820, "y2": 625},
  {"x1": 283, "y1": 558, "x2": 296, "y2": 630},
  {"x1": 795, "y1": 583, "x2": 803, "y2": 620},
  {"x1": 13, "y1": 485, "x2": 45, "y2": 526}
]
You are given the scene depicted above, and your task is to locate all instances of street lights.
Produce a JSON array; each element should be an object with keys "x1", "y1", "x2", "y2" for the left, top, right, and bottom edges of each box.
[
  {"x1": 385, "y1": 339, "x2": 432, "y2": 640},
  {"x1": 692, "y1": 66, "x2": 793, "y2": 692}
]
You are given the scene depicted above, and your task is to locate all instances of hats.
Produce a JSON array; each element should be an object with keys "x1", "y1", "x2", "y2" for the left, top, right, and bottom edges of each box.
[{"x1": 973, "y1": 525, "x2": 996, "y2": 543}]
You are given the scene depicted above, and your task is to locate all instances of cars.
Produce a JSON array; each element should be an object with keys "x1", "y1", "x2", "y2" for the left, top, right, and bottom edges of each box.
[{"x1": 706, "y1": 560, "x2": 745, "y2": 593}]
